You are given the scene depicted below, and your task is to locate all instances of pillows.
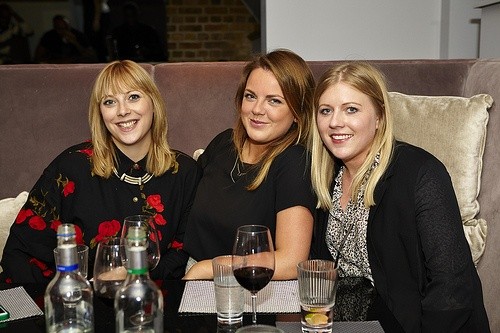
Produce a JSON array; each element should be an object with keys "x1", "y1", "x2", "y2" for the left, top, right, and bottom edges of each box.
[
  {"x1": 0, "y1": 191, "x2": 29, "y2": 262},
  {"x1": 384, "y1": 88, "x2": 494, "y2": 264}
]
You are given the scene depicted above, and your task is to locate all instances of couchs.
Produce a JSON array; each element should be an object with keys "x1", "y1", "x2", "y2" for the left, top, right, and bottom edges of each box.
[{"x1": 0, "y1": 59, "x2": 500, "y2": 333}]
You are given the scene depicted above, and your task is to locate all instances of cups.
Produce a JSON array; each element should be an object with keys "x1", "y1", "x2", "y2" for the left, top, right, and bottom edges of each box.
[
  {"x1": 212, "y1": 255, "x2": 246, "y2": 329},
  {"x1": 297, "y1": 259, "x2": 339, "y2": 333},
  {"x1": 53, "y1": 246, "x2": 88, "y2": 279},
  {"x1": 120, "y1": 215, "x2": 160, "y2": 271},
  {"x1": 93, "y1": 237, "x2": 126, "y2": 301}
]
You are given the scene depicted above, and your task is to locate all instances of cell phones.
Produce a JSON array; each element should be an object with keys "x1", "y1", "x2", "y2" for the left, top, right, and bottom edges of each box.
[{"x1": 0, "y1": 305, "x2": 9, "y2": 320}]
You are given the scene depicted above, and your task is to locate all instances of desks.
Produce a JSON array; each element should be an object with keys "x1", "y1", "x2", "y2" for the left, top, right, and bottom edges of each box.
[{"x1": 0, "y1": 276, "x2": 408, "y2": 333}]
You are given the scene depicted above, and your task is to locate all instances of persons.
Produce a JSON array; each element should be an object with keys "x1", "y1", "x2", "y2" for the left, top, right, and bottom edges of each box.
[
  {"x1": 0, "y1": 58, "x2": 201, "y2": 309},
  {"x1": 172, "y1": 46, "x2": 321, "y2": 285},
  {"x1": 307, "y1": 60, "x2": 492, "y2": 333},
  {"x1": 1, "y1": 4, "x2": 143, "y2": 62}
]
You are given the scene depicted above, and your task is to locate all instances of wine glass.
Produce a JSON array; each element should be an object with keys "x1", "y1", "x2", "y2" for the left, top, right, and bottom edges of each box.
[{"x1": 232, "y1": 224, "x2": 275, "y2": 325}]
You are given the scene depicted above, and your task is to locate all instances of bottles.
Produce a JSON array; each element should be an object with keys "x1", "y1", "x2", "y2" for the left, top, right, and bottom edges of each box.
[
  {"x1": 114, "y1": 246, "x2": 164, "y2": 333},
  {"x1": 45, "y1": 245, "x2": 93, "y2": 333},
  {"x1": 57, "y1": 223, "x2": 75, "y2": 245}
]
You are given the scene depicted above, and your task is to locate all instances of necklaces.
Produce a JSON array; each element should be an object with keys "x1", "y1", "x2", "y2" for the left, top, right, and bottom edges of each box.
[{"x1": 111, "y1": 163, "x2": 157, "y2": 186}]
